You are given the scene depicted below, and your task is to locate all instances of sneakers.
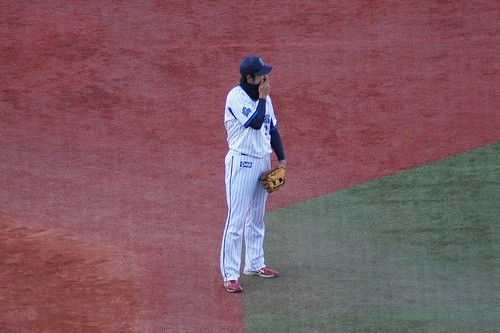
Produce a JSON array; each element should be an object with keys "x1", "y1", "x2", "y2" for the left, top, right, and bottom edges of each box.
[
  {"x1": 223, "y1": 280, "x2": 243, "y2": 292},
  {"x1": 244, "y1": 267, "x2": 278, "y2": 278}
]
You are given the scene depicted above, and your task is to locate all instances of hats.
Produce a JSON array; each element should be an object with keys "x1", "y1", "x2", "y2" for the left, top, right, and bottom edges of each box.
[{"x1": 240, "y1": 55, "x2": 272, "y2": 76}]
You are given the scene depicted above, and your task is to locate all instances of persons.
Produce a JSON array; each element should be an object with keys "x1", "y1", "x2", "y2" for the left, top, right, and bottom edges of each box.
[{"x1": 220, "y1": 56, "x2": 286, "y2": 293}]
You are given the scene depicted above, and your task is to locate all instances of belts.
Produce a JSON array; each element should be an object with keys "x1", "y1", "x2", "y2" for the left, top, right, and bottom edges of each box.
[{"x1": 230, "y1": 149, "x2": 246, "y2": 155}]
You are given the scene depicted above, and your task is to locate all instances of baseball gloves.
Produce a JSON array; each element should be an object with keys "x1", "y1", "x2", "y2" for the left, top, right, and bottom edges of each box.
[{"x1": 259, "y1": 167, "x2": 288, "y2": 194}]
